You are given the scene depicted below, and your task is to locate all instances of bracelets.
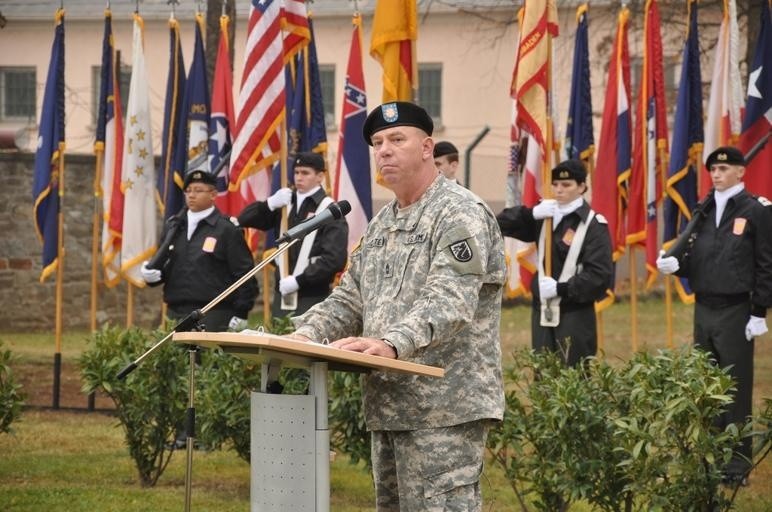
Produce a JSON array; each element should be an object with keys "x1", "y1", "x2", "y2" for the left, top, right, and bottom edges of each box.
[{"x1": 380, "y1": 334, "x2": 397, "y2": 350}]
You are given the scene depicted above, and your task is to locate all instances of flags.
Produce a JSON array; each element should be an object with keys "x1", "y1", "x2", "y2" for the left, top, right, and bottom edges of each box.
[
  {"x1": 508, "y1": 0, "x2": 772, "y2": 311},
  {"x1": 32, "y1": 0, "x2": 419, "y2": 288}
]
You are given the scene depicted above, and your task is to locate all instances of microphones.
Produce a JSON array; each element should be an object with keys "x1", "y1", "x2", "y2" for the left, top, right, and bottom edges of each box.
[{"x1": 275, "y1": 200, "x2": 351, "y2": 245}]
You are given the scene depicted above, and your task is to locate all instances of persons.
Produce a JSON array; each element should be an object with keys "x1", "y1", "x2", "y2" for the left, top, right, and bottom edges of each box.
[
  {"x1": 493, "y1": 159, "x2": 614, "y2": 380},
  {"x1": 287, "y1": 98, "x2": 507, "y2": 512},
  {"x1": 659, "y1": 149, "x2": 770, "y2": 491},
  {"x1": 139, "y1": 170, "x2": 259, "y2": 453},
  {"x1": 432, "y1": 142, "x2": 459, "y2": 180}
]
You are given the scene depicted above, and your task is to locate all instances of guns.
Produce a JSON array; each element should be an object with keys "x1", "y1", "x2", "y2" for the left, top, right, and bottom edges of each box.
[
  {"x1": 145, "y1": 149, "x2": 230, "y2": 270},
  {"x1": 661, "y1": 131, "x2": 771, "y2": 274}
]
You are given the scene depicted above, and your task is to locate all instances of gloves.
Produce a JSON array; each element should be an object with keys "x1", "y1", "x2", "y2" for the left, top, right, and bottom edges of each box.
[
  {"x1": 267, "y1": 187, "x2": 293, "y2": 212},
  {"x1": 746, "y1": 316, "x2": 769, "y2": 342},
  {"x1": 141, "y1": 261, "x2": 161, "y2": 284},
  {"x1": 655, "y1": 249, "x2": 679, "y2": 276},
  {"x1": 532, "y1": 200, "x2": 558, "y2": 221},
  {"x1": 279, "y1": 275, "x2": 299, "y2": 296},
  {"x1": 539, "y1": 276, "x2": 557, "y2": 300},
  {"x1": 229, "y1": 316, "x2": 247, "y2": 331}
]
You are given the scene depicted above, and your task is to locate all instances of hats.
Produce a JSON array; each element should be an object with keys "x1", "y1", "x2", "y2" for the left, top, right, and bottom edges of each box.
[
  {"x1": 552, "y1": 160, "x2": 587, "y2": 181},
  {"x1": 183, "y1": 170, "x2": 218, "y2": 190},
  {"x1": 705, "y1": 147, "x2": 746, "y2": 172},
  {"x1": 363, "y1": 101, "x2": 434, "y2": 146},
  {"x1": 433, "y1": 141, "x2": 458, "y2": 158},
  {"x1": 293, "y1": 151, "x2": 324, "y2": 172}
]
